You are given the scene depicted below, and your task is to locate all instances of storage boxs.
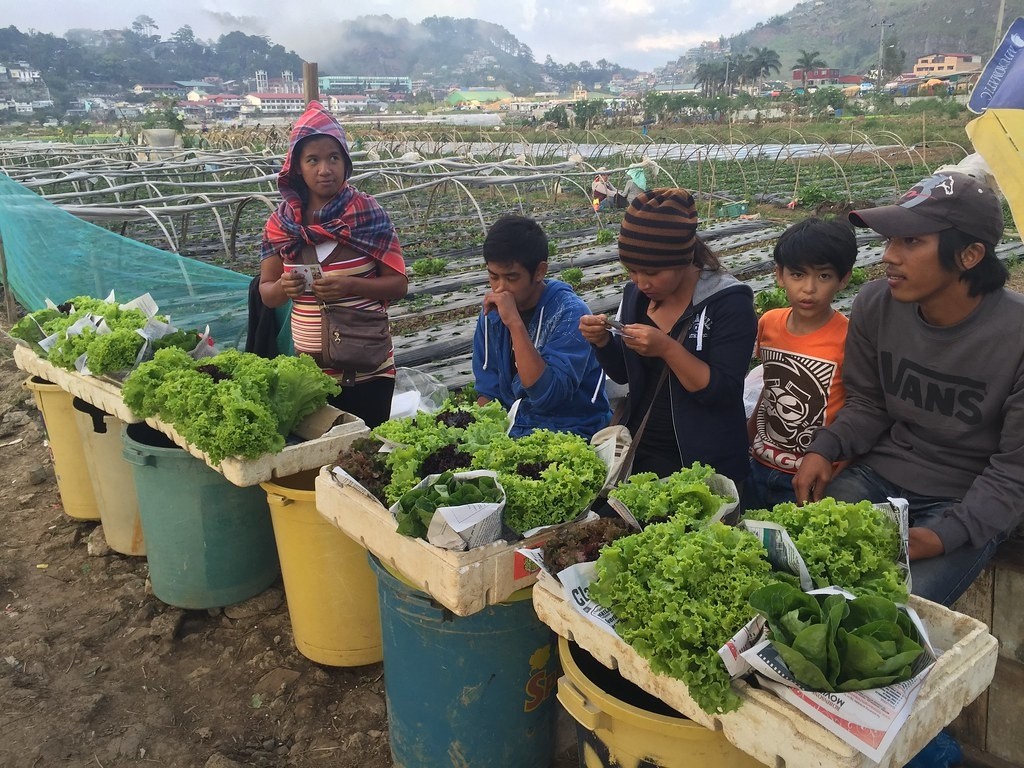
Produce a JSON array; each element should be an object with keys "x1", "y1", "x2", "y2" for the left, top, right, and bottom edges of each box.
[
  {"x1": 531, "y1": 559, "x2": 999, "y2": 768},
  {"x1": 315, "y1": 465, "x2": 598, "y2": 615},
  {"x1": 13, "y1": 344, "x2": 149, "y2": 423},
  {"x1": 145, "y1": 405, "x2": 369, "y2": 489}
]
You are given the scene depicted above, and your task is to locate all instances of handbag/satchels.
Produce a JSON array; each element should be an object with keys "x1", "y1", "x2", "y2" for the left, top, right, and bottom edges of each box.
[
  {"x1": 589, "y1": 425, "x2": 636, "y2": 499},
  {"x1": 320, "y1": 307, "x2": 391, "y2": 373}
]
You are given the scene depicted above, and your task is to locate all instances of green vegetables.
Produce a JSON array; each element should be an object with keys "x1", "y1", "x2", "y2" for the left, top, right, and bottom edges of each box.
[
  {"x1": 393, "y1": 472, "x2": 505, "y2": 542},
  {"x1": 747, "y1": 581, "x2": 924, "y2": 692},
  {"x1": 151, "y1": 328, "x2": 203, "y2": 353}
]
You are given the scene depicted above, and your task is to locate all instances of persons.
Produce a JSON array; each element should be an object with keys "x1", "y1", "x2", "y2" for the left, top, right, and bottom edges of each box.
[
  {"x1": 792, "y1": 168, "x2": 1024, "y2": 768},
  {"x1": 747, "y1": 216, "x2": 850, "y2": 510},
  {"x1": 258, "y1": 99, "x2": 409, "y2": 430},
  {"x1": 471, "y1": 212, "x2": 614, "y2": 447},
  {"x1": 614, "y1": 163, "x2": 646, "y2": 206},
  {"x1": 578, "y1": 189, "x2": 759, "y2": 518},
  {"x1": 591, "y1": 166, "x2": 622, "y2": 208}
]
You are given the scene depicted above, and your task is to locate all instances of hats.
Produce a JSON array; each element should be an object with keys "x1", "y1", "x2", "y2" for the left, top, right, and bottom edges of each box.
[
  {"x1": 625, "y1": 163, "x2": 646, "y2": 191},
  {"x1": 618, "y1": 188, "x2": 698, "y2": 266},
  {"x1": 847, "y1": 171, "x2": 1005, "y2": 245},
  {"x1": 595, "y1": 167, "x2": 611, "y2": 178}
]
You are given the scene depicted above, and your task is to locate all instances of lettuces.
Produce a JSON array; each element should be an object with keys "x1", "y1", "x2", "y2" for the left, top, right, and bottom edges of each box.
[
  {"x1": 454, "y1": 426, "x2": 607, "y2": 533},
  {"x1": 540, "y1": 459, "x2": 803, "y2": 715},
  {"x1": 735, "y1": 495, "x2": 910, "y2": 605},
  {"x1": 9, "y1": 295, "x2": 170, "y2": 377},
  {"x1": 119, "y1": 343, "x2": 341, "y2": 466},
  {"x1": 327, "y1": 398, "x2": 510, "y2": 507}
]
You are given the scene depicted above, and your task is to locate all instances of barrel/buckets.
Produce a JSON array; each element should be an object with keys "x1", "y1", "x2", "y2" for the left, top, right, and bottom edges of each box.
[
  {"x1": 21, "y1": 374, "x2": 102, "y2": 520},
  {"x1": 368, "y1": 549, "x2": 565, "y2": 768},
  {"x1": 258, "y1": 479, "x2": 384, "y2": 667},
  {"x1": 120, "y1": 422, "x2": 279, "y2": 609},
  {"x1": 71, "y1": 396, "x2": 148, "y2": 557},
  {"x1": 556, "y1": 635, "x2": 768, "y2": 767}
]
